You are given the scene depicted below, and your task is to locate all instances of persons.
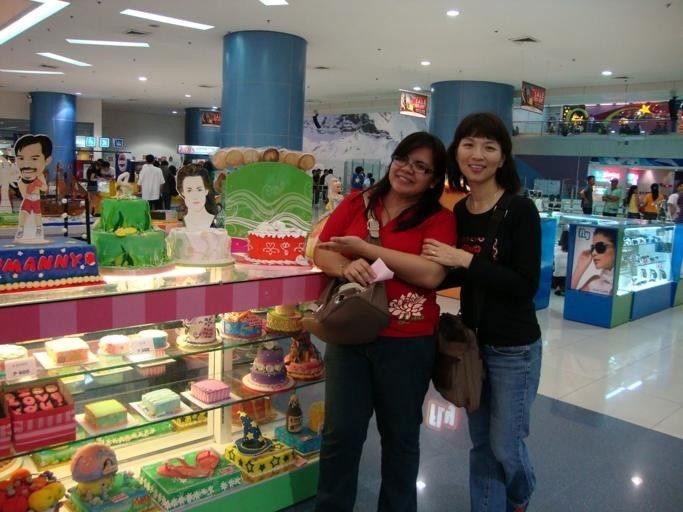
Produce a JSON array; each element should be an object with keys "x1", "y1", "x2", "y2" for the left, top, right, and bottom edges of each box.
[
  {"x1": 551, "y1": 231, "x2": 568, "y2": 295},
  {"x1": 176, "y1": 164, "x2": 223, "y2": 228},
  {"x1": 603, "y1": 179, "x2": 621, "y2": 217},
  {"x1": 579, "y1": 176, "x2": 594, "y2": 214},
  {"x1": 313, "y1": 166, "x2": 375, "y2": 204},
  {"x1": 420, "y1": 112, "x2": 542, "y2": 512},
  {"x1": 13, "y1": 135, "x2": 53, "y2": 244},
  {"x1": 626, "y1": 185, "x2": 642, "y2": 218},
  {"x1": 666, "y1": 182, "x2": 683, "y2": 221},
  {"x1": 642, "y1": 183, "x2": 663, "y2": 220},
  {"x1": 548, "y1": 115, "x2": 669, "y2": 136},
  {"x1": 86, "y1": 154, "x2": 178, "y2": 210},
  {"x1": 312, "y1": 131, "x2": 458, "y2": 512},
  {"x1": 675, "y1": 191, "x2": 683, "y2": 222},
  {"x1": 571, "y1": 227, "x2": 616, "y2": 296}
]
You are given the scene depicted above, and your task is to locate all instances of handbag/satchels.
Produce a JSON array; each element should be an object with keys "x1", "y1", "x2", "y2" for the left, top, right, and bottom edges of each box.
[
  {"x1": 311, "y1": 189, "x2": 391, "y2": 345},
  {"x1": 430, "y1": 309, "x2": 488, "y2": 412}
]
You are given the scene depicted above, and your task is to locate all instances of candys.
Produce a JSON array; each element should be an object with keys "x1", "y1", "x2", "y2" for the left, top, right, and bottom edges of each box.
[{"x1": 209, "y1": 145, "x2": 319, "y2": 176}]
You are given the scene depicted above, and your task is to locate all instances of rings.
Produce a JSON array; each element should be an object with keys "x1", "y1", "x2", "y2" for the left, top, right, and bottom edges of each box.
[{"x1": 431, "y1": 251, "x2": 436, "y2": 256}]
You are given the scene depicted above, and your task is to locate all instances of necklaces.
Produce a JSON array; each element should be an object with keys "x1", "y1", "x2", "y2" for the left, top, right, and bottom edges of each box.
[{"x1": 469, "y1": 187, "x2": 499, "y2": 212}]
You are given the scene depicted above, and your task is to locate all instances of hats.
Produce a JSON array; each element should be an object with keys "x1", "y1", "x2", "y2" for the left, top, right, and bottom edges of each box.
[{"x1": 609, "y1": 179, "x2": 618, "y2": 182}]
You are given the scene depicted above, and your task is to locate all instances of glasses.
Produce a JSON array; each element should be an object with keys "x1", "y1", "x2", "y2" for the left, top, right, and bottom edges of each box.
[
  {"x1": 390, "y1": 154, "x2": 435, "y2": 175},
  {"x1": 591, "y1": 241, "x2": 614, "y2": 254}
]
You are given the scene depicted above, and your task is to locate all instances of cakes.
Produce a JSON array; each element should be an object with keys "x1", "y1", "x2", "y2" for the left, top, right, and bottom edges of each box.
[
  {"x1": 233, "y1": 397, "x2": 276, "y2": 428},
  {"x1": 272, "y1": 395, "x2": 332, "y2": 465},
  {"x1": 180, "y1": 316, "x2": 218, "y2": 348},
  {"x1": 85, "y1": 399, "x2": 129, "y2": 430},
  {"x1": 138, "y1": 329, "x2": 168, "y2": 349},
  {"x1": 190, "y1": 378, "x2": 229, "y2": 403},
  {"x1": 0, "y1": 234, "x2": 108, "y2": 296},
  {"x1": 165, "y1": 228, "x2": 236, "y2": 267},
  {"x1": 242, "y1": 341, "x2": 296, "y2": 391},
  {"x1": 281, "y1": 335, "x2": 325, "y2": 383},
  {"x1": 141, "y1": 388, "x2": 183, "y2": 421},
  {"x1": 270, "y1": 303, "x2": 305, "y2": 334},
  {"x1": 140, "y1": 446, "x2": 243, "y2": 511},
  {"x1": 0, "y1": 342, "x2": 28, "y2": 384},
  {"x1": 44, "y1": 335, "x2": 90, "y2": 364},
  {"x1": 136, "y1": 363, "x2": 168, "y2": 377},
  {"x1": 6, "y1": 379, "x2": 78, "y2": 451},
  {"x1": 224, "y1": 311, "x2": 262, "y2": 341},
  {"x1": 0, "y1": 457, "x2": 26, "y2": 484},
  {"x1": 224, "y1": 412, "x2": 297, "y2": 483},
  {"x1": 92, "y1": 371, "x2": 123, "y2": 386},
  {"x1": 59, "y1": 376, "x2": 85, "y2": 396},
  {"x1": 90, "y1": 173, "x2": 175, "y2": 274},
  {"x1": 63, "y1": 444, "x2": 154, "y2": 512},
  {"x1": 30, "y1": 424, "x2": 97, "y2": 472},
  {"x1": 244, "y1": 220, "x2": 309, "y2": 267},
  {"x1": 172, "y1": 408, "x2": 208, "y2": 431},
  {"x1": 97, "y1": 420, "x2": 173, "y2": 448},
  {"x1": 97, "y1": 329, "x2": 131, "y2": 357}
]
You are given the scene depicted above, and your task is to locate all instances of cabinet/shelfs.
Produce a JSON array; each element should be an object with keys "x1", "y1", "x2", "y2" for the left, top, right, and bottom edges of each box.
[
  {"x1": 0, "y1": 263, "x2": 325, "y2": 508},
  {"x1": 561, "y1": 225, "x2": 676, "y2": 329}
]
